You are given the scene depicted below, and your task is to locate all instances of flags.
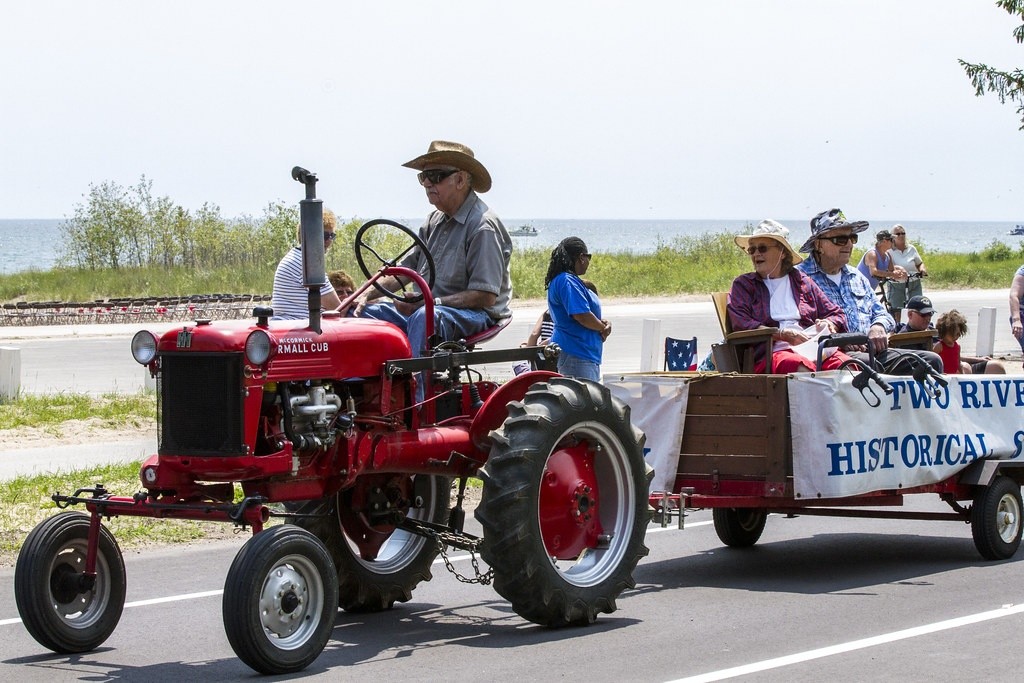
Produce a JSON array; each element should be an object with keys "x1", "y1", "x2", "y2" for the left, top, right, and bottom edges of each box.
[{"x1": 667, "y1": 337, "x2": 698, "y2": 371}]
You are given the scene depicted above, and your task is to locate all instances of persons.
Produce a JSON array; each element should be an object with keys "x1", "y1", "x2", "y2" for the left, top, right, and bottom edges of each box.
[
  {"x1": 798, "y1": 209, "x2": 944, "y2": 376},
  {"x1": 545, "y1": 236, "x2": 612, "y2": 383},
  {"x1": 898, "y1": 296, "x2": 1006, "y2": 374},
  {"x1": 342, "y1": 140, "x2": 513, "y2": 412},
  {"x1": 886, "y1": 224, "x2": 926, "y2": 323},
  {"x1": 329, "y1": 272, "x2": 357, "y2": 302},
  {"x1": 933, "y1": 310, "x2": 967, "y2": 374},
  {"x1": 726, "y1": 219, "x2": 858, "y2": 374},
  {"x1": 1009, "y1": 264, "x2": 1024, "y2": 370},
  {"x1": 856, "y1": 229, "x2": 904, "y2": 292},
  {"x1": 526, "y1": 310, "x2": 558, "y2": 372},
  {"x1": 272, "y1": 210, "x2": 341, "y2": 320}
]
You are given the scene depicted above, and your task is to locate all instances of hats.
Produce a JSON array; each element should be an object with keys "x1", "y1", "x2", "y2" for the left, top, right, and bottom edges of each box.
[
  {"x1": 401, "y1": 141, "x2": 491, "y2": 194},
  {"x1": 734, "y1": 219, "x2": 804, "y2": 266},
  {"x1": 799, "y1": 208, "x2": 869, "y2": 253},
  {"x1": 906, "y1": 295, "x2": 937, "y2": 314},
  {"x1": 876, "y1": 230, "x2": 898, "y2": 241}
]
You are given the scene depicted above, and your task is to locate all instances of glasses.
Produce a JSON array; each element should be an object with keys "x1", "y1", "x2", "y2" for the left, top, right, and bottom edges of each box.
[
  {"x1": 912, "y1": 311, "x2": 934, "y2": 317},
  {"x1": 747, "y1": 245, "x2": 782, "y2": 255},
  {"x1": 324, "y1": 231, "x2": 338, "y2": 241},
  {"x1": 335, "y1": 290, "x2": 354, "y2": 296},
  {"x1": 894, "y1": 232, "x2": 905, "y2": 236},
  {"x1": 417, "y1": 169, "x2": 463, "y2": 186},
  {"x1": 886, "y1": 239, "x2": 893, "y2": 242},
  {"x1": 577, "y1": 253, "x2": 592, "y2": 261},
  {"x1": 817, "y1": 232, "x2": 858, "y2": 246}
]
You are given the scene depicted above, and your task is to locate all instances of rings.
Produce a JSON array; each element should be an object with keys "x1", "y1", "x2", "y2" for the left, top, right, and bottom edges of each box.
[
  {"x1": 607, "y1": 332, "x2": 609, "y2": 335},
  {"x1": 848, "y1": 346, "x2": 853, "y2": 349}
]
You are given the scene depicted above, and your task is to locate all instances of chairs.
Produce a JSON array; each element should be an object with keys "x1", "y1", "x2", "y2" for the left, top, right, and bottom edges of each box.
[{"x1": 464, "y1": 314, "x2": 513, "y2": 347}]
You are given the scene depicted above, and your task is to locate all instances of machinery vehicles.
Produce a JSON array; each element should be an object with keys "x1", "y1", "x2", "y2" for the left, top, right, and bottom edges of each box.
[{"x1": 11, "y1": 164, "x2": 685, "y2": 676}]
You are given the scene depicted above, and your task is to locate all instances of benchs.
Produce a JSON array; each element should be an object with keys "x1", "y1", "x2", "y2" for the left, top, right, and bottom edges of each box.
[{"x1": 712, "y1": 291, "x2": 939, "y2": 376}]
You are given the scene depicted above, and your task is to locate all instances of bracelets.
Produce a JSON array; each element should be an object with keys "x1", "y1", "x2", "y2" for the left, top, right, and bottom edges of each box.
[{"x1": 780, "y1": 329, "x2": 783, "y2": 338}]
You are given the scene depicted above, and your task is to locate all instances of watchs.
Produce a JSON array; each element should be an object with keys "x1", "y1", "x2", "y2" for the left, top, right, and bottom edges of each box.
[{"x1": 435, "y1": 298, "x2": 442, "y2": 306}]
